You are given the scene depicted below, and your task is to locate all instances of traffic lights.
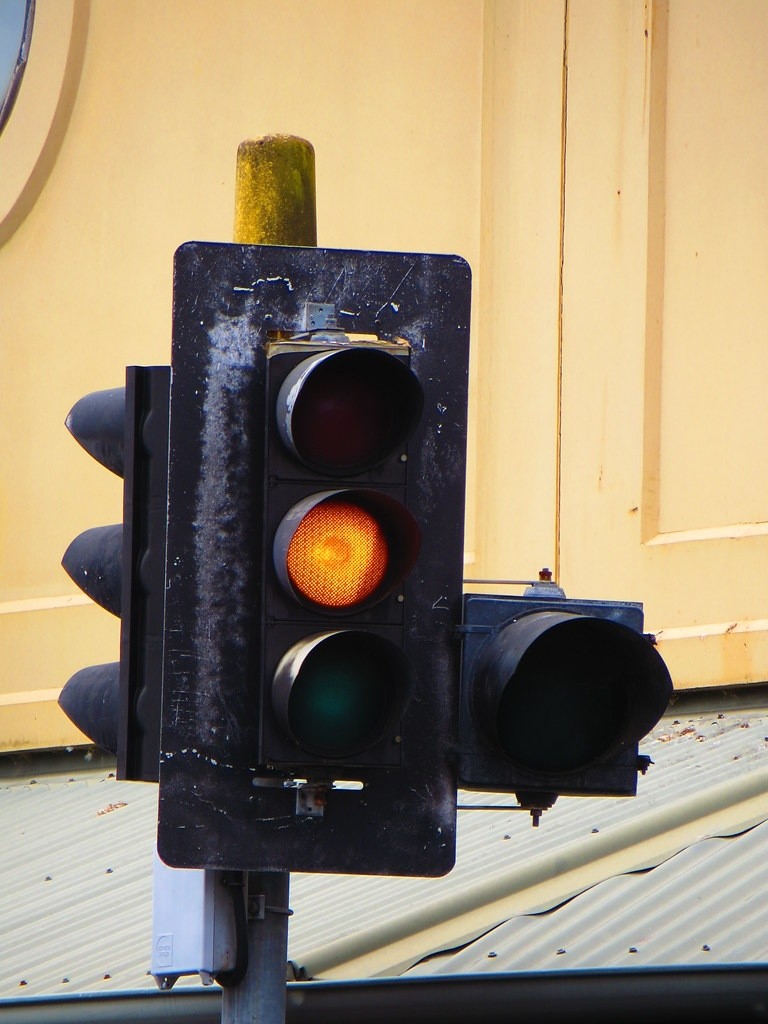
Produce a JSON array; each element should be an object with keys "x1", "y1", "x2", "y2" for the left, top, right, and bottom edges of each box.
[
  {"x1": 459, "y1": 590, "x2": 675, "y2": 801},
  {"x1": 154, "y1": 235, "x2": 476, "y2": 880},
  {"x1": 59, "y1": 363, "x2": 168, "y2": 784}
]
